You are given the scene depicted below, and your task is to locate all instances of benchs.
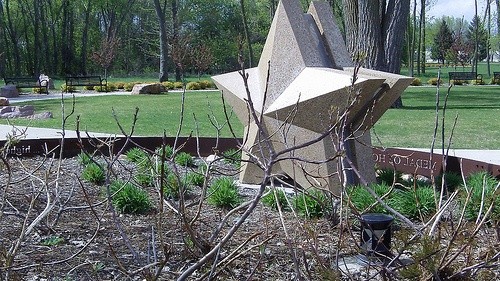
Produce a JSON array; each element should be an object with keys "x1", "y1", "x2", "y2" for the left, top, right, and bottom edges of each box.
[
  {"x1": 63, "y1": 76, "x2": 108, "y2": 93},
  {"x1": 492, "y1": 71, "x2": 500, "y2": 84},
  {"x1": 4, "y1": 77, "x2": 49, "y2": 96},
  {"x1": 448, "y1": 72, "x2": 483, "y2": 86}
]
